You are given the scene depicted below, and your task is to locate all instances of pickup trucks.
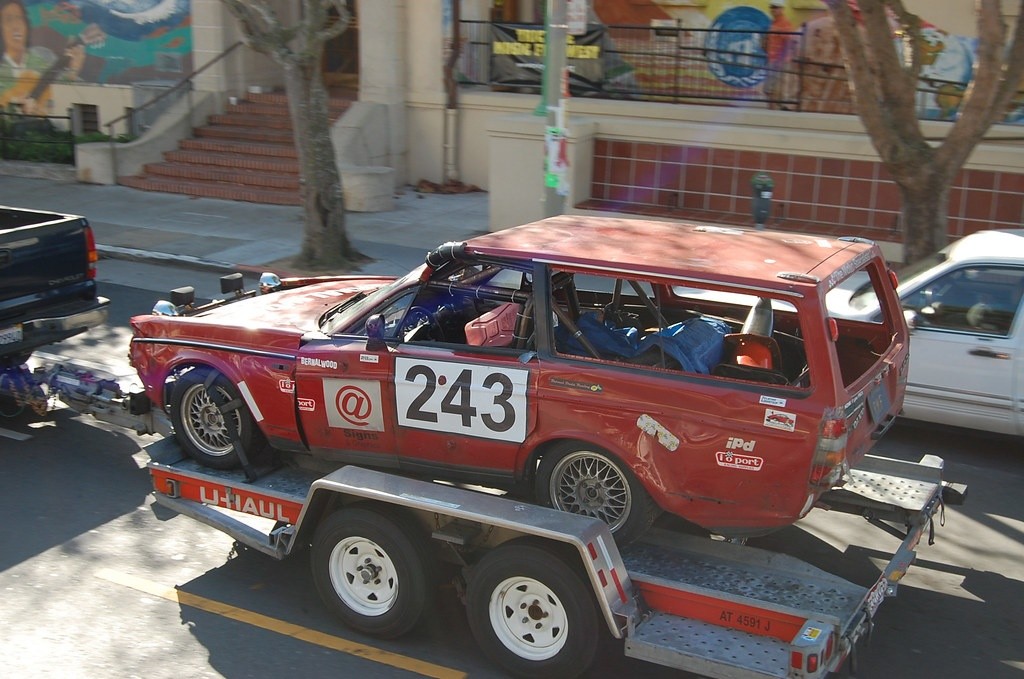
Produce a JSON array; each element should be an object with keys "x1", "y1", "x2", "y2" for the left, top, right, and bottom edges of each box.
[{"x1": 0, "y1": 203, "x2": 110, "y2": 363}]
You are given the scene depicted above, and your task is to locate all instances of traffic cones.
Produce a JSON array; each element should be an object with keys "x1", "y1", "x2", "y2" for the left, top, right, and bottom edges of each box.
[{"x1": 713, "y1": 339, "x2": 788, "y2": 385}]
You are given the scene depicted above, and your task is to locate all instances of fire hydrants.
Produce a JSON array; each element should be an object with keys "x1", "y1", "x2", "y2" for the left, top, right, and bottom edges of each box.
[{"x1": 750, "y1": 172, "x2": 774, "y2": 228}]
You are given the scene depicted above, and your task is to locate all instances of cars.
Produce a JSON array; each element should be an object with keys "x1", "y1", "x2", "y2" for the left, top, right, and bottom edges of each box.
[{"x1": 702, "y1": 228, "x2": 1024, "y2": 437}]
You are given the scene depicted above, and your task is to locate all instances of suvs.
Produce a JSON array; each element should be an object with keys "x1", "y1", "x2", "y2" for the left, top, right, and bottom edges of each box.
[{"x1": 127, "y1": 213, "x2": 911, "y2": 546}]
